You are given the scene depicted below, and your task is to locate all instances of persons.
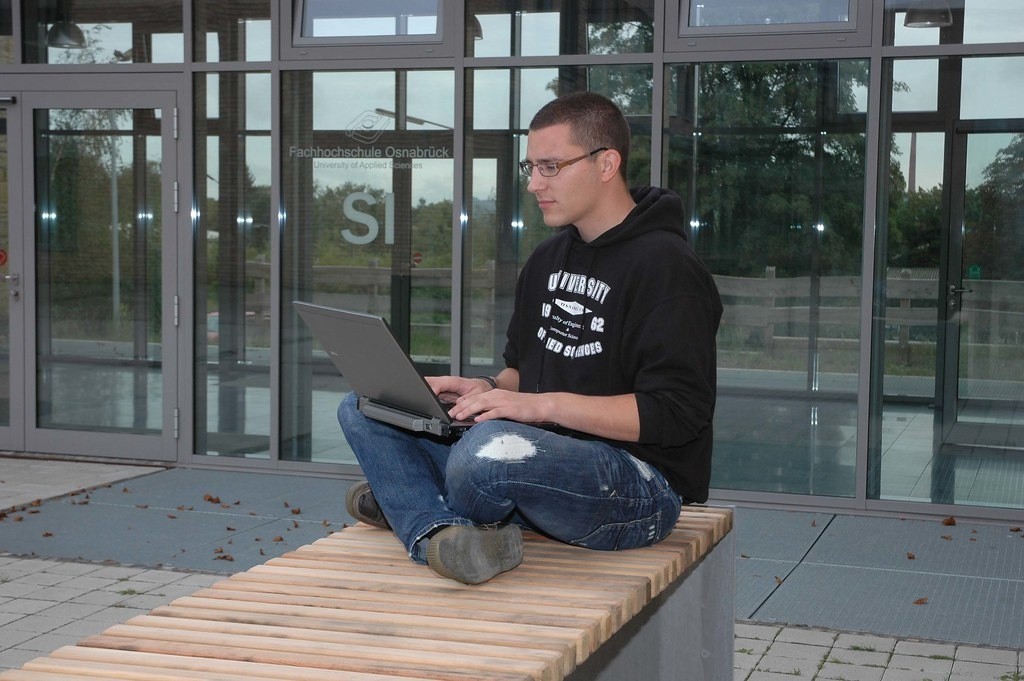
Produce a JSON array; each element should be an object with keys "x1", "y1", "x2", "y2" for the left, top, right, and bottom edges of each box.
[{"x1": 336, "y1": 91, "x2": 723, "y2": 585}]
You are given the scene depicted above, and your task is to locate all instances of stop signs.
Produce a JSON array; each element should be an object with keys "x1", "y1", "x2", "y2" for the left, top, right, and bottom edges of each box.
[{"x1": 413, "y1": 254, "x2": 422, "y2": 264}]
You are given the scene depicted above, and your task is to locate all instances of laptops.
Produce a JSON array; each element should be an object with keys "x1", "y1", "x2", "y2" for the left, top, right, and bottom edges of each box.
[{"x1": 291, "y1": 299, "x2": 560, "y2": 442}]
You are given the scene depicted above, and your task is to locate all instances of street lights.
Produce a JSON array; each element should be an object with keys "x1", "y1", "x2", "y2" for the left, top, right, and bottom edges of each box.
[{"x1": 110, "y1": 48, "x2": 134, "y2": 335}]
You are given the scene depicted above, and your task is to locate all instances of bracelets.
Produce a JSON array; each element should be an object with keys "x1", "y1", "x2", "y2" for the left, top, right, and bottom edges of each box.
[{"x1": 476, "y1": 376, "x2": 497, "y2": 388}]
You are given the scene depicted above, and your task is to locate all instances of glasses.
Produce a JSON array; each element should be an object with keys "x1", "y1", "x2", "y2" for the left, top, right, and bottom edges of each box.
[{"x1": 517, "y1": 147, "x2": 609, "y2": 177}]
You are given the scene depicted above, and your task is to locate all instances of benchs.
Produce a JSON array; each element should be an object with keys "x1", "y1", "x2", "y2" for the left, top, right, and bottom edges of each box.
[{"x1": 0, "y1": 502, "x2": 737, "y2": 681}]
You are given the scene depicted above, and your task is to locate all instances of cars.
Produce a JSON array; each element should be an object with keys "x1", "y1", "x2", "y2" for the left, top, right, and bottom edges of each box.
[{"x1": 208, "y1": 311, "x2": 270, "y2": 346}]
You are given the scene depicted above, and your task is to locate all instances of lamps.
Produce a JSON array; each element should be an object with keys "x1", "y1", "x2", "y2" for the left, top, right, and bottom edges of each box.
[
  {"x1": 904, "y1": 0, "x2": 953, "y2": 28},
  {"x1": 42, "y1": 0, "x2": 86, "y2": 49},
  {"x1": 473, "y1": 15, "x2": 483, "y2": 39}
]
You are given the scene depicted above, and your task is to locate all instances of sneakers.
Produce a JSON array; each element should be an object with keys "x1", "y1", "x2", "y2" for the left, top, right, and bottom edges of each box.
[
  {"x1": 424, "y1": 520, "x2": 525, "y2": 584},
  {"x1": 344, "y1": 479, "x2": 392, "y2": 531}
]
